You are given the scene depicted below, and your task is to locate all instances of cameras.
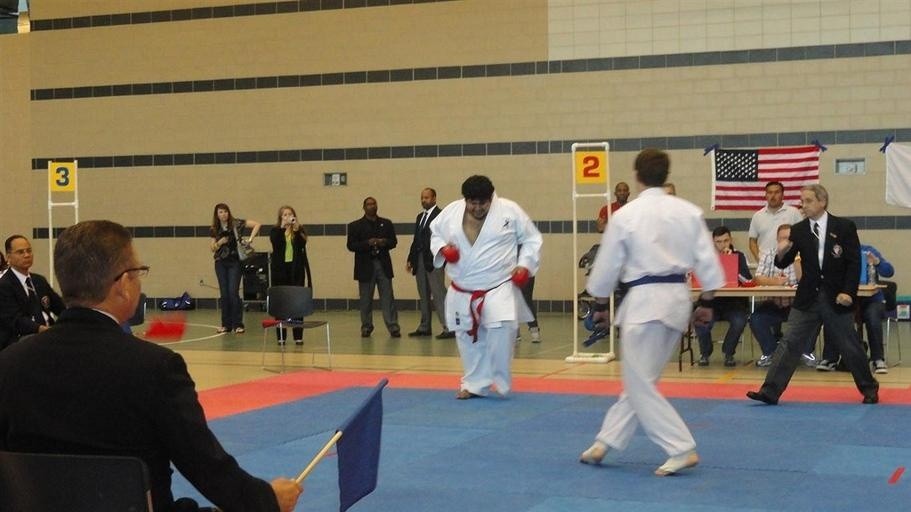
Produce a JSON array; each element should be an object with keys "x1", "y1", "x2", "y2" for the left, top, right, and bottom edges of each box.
[{"x1": 286, "y1": 216, "x2": 296, "y2": 221}]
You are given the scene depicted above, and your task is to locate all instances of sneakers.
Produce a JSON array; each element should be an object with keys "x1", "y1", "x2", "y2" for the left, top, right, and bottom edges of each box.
[
  {"x1": 756, "y1": 356, "x2": 772, "y2": 366},
  {"x1": 217, "y1": 326, "x2": 231, "y2": 332},
  {"x1": 802, "y1": 354, "x2": 817, "y2": 366},
  {"x1": 531, "y1": 327, "x2": 541, "y2": 343},
  {"x1": 816, "y1": 359, "x2": 836, "y2": 371},
  {"x1": 875, "y1": 359, "x2": 888, "y2": 374},
  {"x1": 725, "y1": 356, "x2": 735, "y2": 366},
  {"x1": 236, "y1": 327, "x2": 244, "y2": 333},
  {"x1": 700, "y1": 357, "x2": 709, "y2": 365}
]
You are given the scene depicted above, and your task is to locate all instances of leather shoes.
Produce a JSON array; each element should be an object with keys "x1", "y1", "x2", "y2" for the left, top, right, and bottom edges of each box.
[
  {"x1": 436, "y1": 331, "x2": 455, "y2": 338},
  {"x1": 409, "y1": 331, "x2": 432, "y2": 336},
  {"x1": 863, "y1": 381, "x2": 879, "y2": 404},
  {"x1": 362, "y1": 330, "x2": 370, "y2": 337},
  {"x1": 746, "y1": 391, "x2": 778, "y2": 405},
  {"x1": 392, "y1": 331, "x2": 400, "y2": 337},
  {"x1": 297, "y1": 339, "x2": 303, "y2": 345},
  {"x1": 279, "y1": 340, "x2": 285, "y2": 345}
]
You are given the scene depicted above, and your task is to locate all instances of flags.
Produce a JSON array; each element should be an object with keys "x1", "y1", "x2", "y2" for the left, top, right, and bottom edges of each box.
[
  {"x1": 706, "y1": 142, "x2": 826, "y2": 216},
  {"x1": 337, "y1": 379, "x2": 391, "y2": 511}
]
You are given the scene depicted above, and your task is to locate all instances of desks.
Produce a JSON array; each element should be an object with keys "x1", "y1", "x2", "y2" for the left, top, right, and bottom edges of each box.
[{"x1": 687, "y1": 277, "x2": 880, "y2": 369}]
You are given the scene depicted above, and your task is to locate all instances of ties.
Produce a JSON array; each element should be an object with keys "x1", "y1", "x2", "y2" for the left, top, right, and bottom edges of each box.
[
  {"x1": 25, "y1": 277, "x2": 46, "y2": 326},
  {"x1": 813, "y1": 223, "x2": 819, "y2": 252},
  {"x1": 421, "y1": 212, "x2": 428, "y2": 229}
]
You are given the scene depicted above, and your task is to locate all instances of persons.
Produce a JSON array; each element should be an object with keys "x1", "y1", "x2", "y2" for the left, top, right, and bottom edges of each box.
[
  {"x1": 271, "y1": 207, "x2": 309, "y2": 350},
  {"x1": 432, "y1": 173, "x2": 543, "y2": 401},
  {"x1": 346, "y1": 198, "x2": 402, "y2": 336},
  {"x1": 582, "y1": 151, "x2": 725, "y2": 472},
  {"x1": 847, "y1": 245, "x2": 895, "y2": 375},
  {"x1": 749, "y1": 181, "x2": 807, "y2": 261},
  {"x1": 408, "y1": 189, "x2": 458, "y2": 337},
  {"x1": 692, "y1": 225, "x2": 753, "y2": 369},
  {"x1": 210, "y1": 205, "x2": 260, "y2": 332},
  {"x1": 3, "y1": 220, "x2": 302, "y2": 510},
  {"x1": 751, "y1": 226, "x2": 816, "y2": 368},
  {"x1": 597, "y1": 183, "x2": 628, "y2": 231},
  {"x1": 2, "y1": 235, "x2": 64, "y2": 345},
  {"x1": 746, "y1": 185, "x2": 880, "y2": 405}
]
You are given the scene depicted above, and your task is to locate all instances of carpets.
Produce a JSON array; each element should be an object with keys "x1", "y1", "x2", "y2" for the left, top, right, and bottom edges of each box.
[{"x1": 168, "y1": 371, "x2": 911, "y2": 512}]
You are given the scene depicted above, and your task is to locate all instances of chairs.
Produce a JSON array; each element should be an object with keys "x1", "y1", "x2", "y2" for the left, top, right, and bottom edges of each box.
[
  {"x1": 870, "y1": 274, "x2": 903, "y2": 370},
  {"x1": 734, "y1": 322, "x2": 756, "y2": 364},
  {"x1": 0, "y1": 447, "x2": 154, "y2": 512},
  {"x1": 258, "y1": 285, "x2": 336, "y2": 380}
]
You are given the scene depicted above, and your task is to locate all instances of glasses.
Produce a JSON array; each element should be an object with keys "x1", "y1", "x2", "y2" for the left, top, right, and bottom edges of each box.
[
  {"x1": 7, "y1": 248, "x2": 32, "y2": 254},
  {"x1": 114, "y1": 265, "x2": 150, "y2": 281}
]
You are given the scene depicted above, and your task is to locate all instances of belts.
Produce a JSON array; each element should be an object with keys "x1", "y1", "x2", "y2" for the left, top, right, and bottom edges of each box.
[
  {"x1": 626, "y1": 275, "x2": 686, "y2": 285},
  {"x1": 451, "y1": 277, "x2": 513, "y2": 343}
]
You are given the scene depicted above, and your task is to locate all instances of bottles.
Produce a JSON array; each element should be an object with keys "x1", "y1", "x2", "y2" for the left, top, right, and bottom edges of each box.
[{"x1": 868, "y1": 260, "x2": 877, "y2": 286}]
